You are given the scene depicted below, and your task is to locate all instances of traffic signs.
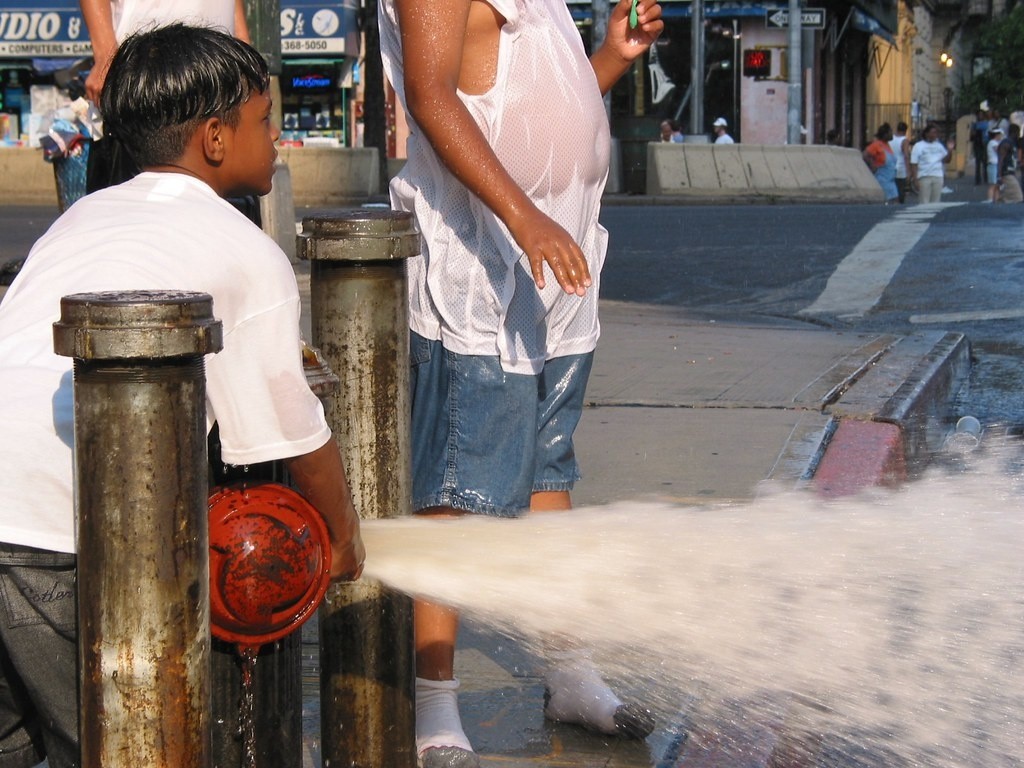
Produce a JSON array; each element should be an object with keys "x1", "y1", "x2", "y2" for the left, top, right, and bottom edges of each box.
[{"x1": 764, "y1": 7, "x2": 825, "y2": 32}]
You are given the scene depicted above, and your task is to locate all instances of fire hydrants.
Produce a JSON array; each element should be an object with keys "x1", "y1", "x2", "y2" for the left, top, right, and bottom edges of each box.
[{"x1": 206, "y1": 478, "x2": 333, "y2": 668}]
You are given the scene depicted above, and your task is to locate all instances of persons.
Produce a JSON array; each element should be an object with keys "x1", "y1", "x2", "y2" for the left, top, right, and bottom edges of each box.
[
  {"x1": 379, "y1": 0, "x2": 664, "y2": 768},
  {"x1": 660, "y1": 120, "x2": 684, "y2": 143},
  {"x1": 713, "y1": 118, "x2": 735, "y2": 145},
  {"x1": 969, "y1": 101, "x2": 1024, "y2": 204},
  {"x1": 0, "y1": 19, "x2": 366, "y2": 768},
  {"x1": 85, "y1": 0, "x2": 262, "y2": 231},
  {"x1": 826, "y1": 121, "x2": 955, "y2": 203}
]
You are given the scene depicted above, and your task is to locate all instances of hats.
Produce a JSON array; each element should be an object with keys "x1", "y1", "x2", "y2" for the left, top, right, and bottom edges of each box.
[{"x1": 713, "y1": 117, "x2": 727, "y2": 127}]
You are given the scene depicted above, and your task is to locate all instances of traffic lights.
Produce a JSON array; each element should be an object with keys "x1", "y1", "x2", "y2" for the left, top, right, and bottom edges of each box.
[{"x1": 743, "y1": 48, "x2": 771, "y2": 79}]
